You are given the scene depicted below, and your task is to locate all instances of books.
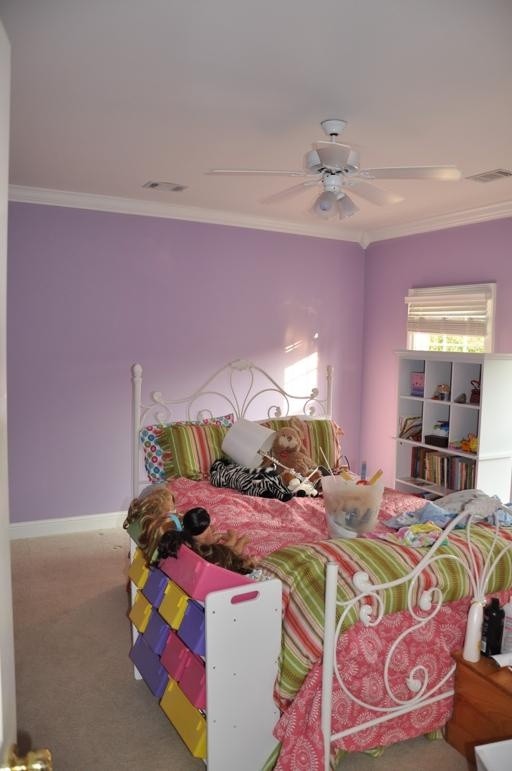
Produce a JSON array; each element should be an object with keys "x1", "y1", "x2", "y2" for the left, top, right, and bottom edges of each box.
[
  {"x1": 398, "y1": 415, "x2": 422, "y2": 439},
  {"x1": 410, "y1": 446, "x2": 476, "y2": 491}
]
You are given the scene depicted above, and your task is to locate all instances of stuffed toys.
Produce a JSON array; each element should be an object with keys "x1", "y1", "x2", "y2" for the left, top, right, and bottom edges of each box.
[{"x1": 260, "y1": 428, "x2": 321, "y2": 486}]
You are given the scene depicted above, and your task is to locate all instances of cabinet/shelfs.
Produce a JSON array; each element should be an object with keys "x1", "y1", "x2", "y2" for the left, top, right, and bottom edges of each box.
[
  {"x1": 443, "y1": 647, "x2": 512, "y2": 766},
  {"x1": 129, "y1": 515, "x2": 283, "y2": 771},
  {"x1": 390, "y1": 348, "x2": 511, "y2": 505}
]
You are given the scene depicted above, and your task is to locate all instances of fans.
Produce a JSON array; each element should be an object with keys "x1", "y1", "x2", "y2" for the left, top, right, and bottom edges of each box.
[{"x1": 203, "y1": 120, "x2": 462, "y2": 210}]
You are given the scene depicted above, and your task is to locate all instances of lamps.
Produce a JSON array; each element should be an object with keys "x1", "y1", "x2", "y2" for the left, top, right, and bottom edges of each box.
[
  {"x1": 221, "y1": 417, "x2": 319, "y2": 494},
  {"x1": 310, "y1": 189, "x2": 363, "y2": 223}
]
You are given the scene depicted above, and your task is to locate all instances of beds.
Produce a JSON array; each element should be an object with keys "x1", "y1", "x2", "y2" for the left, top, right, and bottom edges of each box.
[{"x1": 130, "y1": 359, "x2": 512, "y2": 771}]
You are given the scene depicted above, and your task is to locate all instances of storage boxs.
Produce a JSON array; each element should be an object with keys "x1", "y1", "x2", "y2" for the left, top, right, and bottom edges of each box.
[
  {"x1": 408, "y1": 372, "x2": 424, "y2": 397},
  {"x1": 129, "y1": 536, "x2": 258, "y2": 758},
  {"x1": 474, "y1": 740, "x2": 512, "y2": 770}
]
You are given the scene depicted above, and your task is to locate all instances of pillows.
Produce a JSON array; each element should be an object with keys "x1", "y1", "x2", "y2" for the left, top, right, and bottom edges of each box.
[
  {"x1": 261, "y1": 417, "x2": 344, "y2": 474},
  {"x1": 139, "y1": 413, "x2": 234, "y2": 483},
  {"x1": 156, "y1": 421, "x2": 231, "y2": 481}
]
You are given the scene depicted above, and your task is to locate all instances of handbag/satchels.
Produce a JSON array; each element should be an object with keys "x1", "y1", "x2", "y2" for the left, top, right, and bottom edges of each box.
[{"x1": 470, "y1": 379, "x2": 480, "y2": 405}]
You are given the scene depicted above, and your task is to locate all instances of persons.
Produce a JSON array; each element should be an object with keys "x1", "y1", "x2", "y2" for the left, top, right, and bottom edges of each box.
[{"x1": 123, "y1": 486, "x2": 258, "y2": 574}]
